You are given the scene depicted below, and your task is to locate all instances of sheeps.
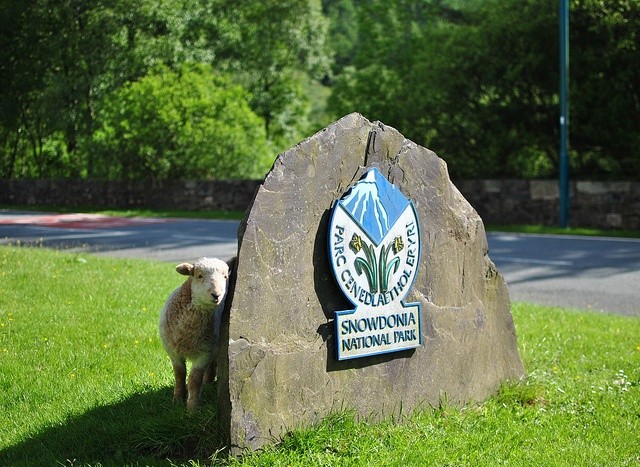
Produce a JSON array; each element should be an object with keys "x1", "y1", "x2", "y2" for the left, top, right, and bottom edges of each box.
[{"x1": 158, "y1": 256, "x2": 236, "y2": 419}]
